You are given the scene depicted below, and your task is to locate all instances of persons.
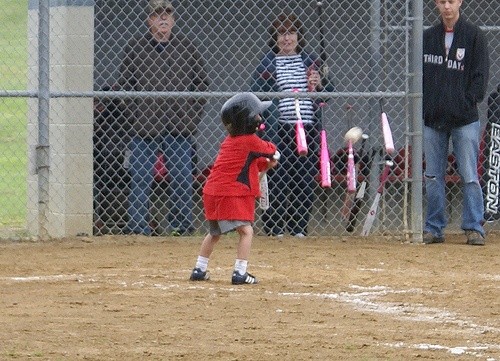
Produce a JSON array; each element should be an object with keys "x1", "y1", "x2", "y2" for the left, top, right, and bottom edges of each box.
[
  {"x1": 250, "y1": 15, "x2": 334, "y2": 238},
  {"x1": 189, "y1": 91, "x2": 280, "y2": 285},
  {"x1": 423, "y1": 0, "x2": 489, "y2": 246},
  {"x1": 119, "y1": 0, "x2": 211, "y2": 236}
]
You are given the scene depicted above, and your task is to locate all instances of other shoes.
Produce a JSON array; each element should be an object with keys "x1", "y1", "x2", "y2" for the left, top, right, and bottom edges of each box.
[{"x1": 171, "y1": 229, "x2": 194, "y2": 237}]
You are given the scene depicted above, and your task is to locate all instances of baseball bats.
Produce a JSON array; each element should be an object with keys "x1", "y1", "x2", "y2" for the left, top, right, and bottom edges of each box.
[
  {"x1": 342, "y1": 134, "x2": 369, "y2": 221},
  {"x1": 361, "y1": 160, "x2": 394, "y2": 236},
  {"x1": 258, "y1": 124, "x2": 270, "y2": 210},
  {"x1": 258, "y1": 151, "x2": 281, "y2": 181},
  {"x1": 346, "y1": 105, "x2": 356, "y2": 192},
  {"x1": 379, "y1": 91, "x2": 394, "y2": 153},
  {"x1": 346, "y1": 147, "x2": 378, "y2": 232},
  {"x1": 319, "y1": 103, "x2": 332, "y2": 188},
  {"x1": 293, "y1": 90, "x2": 308, "y2": 155}
]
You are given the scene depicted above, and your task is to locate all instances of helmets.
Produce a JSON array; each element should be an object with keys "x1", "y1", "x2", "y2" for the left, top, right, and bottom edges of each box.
[{"x1": 220, "y1": 92, "x2": 273, "y2": 129}]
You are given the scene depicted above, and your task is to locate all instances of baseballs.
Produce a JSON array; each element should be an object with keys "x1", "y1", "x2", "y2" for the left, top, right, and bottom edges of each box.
[{"x1": 347, "y1": 128, "x2": 362, "y2": 142}]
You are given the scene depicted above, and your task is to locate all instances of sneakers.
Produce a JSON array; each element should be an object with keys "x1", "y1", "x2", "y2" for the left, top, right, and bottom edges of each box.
[
  {"x1": 189, "y1": 267, "x2": 211, "y2": 281},
  {"x1": 464, "y1": 229, "x2": 486, "y2": 245},
  {"x1": 423, "y1": 231, "x2": 444, "y2": 243},
  {"x1": 232, "y1": 270, "x2": 258, "y2": 284}
]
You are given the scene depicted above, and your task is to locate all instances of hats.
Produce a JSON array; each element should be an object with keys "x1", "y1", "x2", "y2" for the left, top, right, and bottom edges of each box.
[{"x1": 148, "y1": 0, "x2": 175, "y2": 14}]
